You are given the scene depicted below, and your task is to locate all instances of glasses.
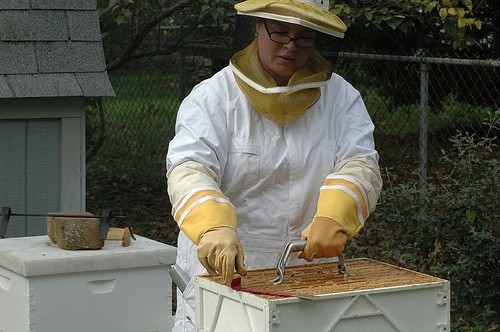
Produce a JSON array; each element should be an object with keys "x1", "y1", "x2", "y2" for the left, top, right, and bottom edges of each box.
[{"x1": 263, "y1": 19, "x2": 319, "y2": 48}]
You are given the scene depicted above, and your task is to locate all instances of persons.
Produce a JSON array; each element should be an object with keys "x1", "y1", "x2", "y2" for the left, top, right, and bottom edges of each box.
[{"x1": 166, "y1": 0, "x2": 382, "y2": 332}]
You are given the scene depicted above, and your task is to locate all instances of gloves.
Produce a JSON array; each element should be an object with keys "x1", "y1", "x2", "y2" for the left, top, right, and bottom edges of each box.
[
  {"x1": 297, "y1": 217, "x2": 347, "y2": 262},
  {"x1": 196, "y1": 227, "x2": 247, "y2": 286}
]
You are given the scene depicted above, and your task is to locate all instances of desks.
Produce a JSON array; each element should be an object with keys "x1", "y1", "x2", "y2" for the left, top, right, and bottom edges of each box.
[{"x1": 0, "y1": 233, "x2": 178, "y2": 332}]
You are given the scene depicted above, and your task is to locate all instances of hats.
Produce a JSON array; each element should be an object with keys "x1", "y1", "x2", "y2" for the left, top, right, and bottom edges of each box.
[{"x1": 233, "y1": 0, "x2": 346, "y2": 39}]
